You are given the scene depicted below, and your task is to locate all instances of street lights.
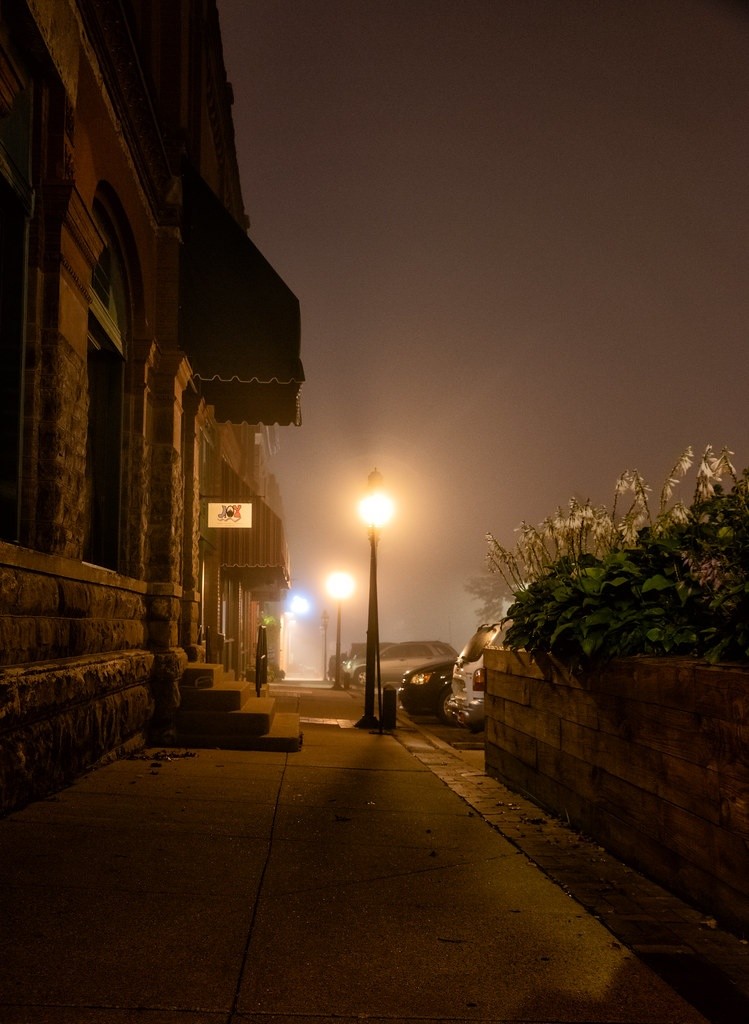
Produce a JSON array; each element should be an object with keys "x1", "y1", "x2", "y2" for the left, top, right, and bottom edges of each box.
[
  {"x1": 354, "y1": 491, "x2": 395, "y2": 731},
  {"x1": 329, "y1": 571, "x2": 352, "y2": 690},
  {"x1": 321, "y1": 608, "x2": 329, "y2": 681}
]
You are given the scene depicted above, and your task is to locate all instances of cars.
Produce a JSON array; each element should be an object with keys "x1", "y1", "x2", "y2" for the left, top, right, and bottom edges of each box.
[
  {"x1": 397, "y1": 656, "x2": 460, "y2": 721},
  {"x1": 330, "y1": 642, "x2": 398, "y2": 687}
]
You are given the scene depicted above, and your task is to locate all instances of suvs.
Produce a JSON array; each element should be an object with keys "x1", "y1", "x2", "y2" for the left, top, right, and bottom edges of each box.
[
  {"x1": 347, "y1": 640, "x2": 461, "y2": 689},
  {"x1": 444, "y1": 616, "x2": 521, "y2": 733}
]
